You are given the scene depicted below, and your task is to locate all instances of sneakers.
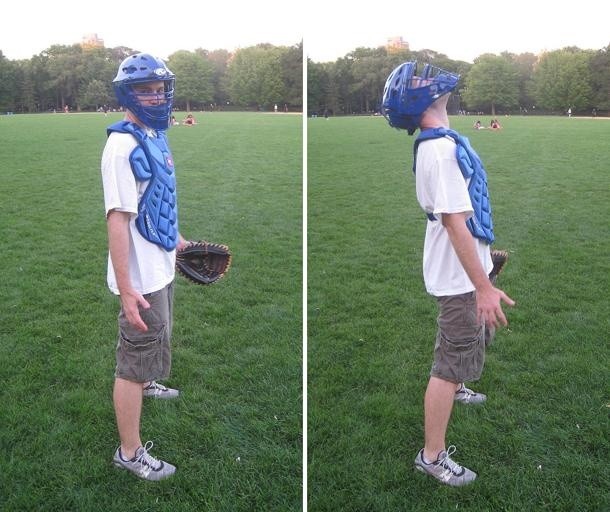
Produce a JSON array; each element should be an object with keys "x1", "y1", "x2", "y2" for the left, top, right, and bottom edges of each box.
[
  {"x1": 144, "y1": 381, "x2": 179, "y2": 398},
  {"x1": 414, "y1": 445, "x2": 478, "y2": 487},
  {"x1": 454, "y1": 383, "x2": 487, "y2": 403},
  {"x1": 112, "y1": 441, "x2": 176, "y2": 481}
]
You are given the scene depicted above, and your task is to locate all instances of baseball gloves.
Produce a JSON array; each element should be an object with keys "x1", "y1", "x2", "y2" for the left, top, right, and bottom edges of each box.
[
  {"x1": 176, "y1": 242, "x2": 231, "y2": 284},
  {"x1": 489, "y1": 252, "x2": 507, "y2": 284}
]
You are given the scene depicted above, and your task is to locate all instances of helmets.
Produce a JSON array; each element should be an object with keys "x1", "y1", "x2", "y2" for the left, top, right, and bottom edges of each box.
[
  {"x1": 112, "y1": 54, "x2": 175, "y2": 130},
  {"x1": 379, "y1": 60, "x2": 461, "y2": 134}
]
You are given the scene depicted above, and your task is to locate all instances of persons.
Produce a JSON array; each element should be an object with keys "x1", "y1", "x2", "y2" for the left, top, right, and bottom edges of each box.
[
  {"x1": 379, "y1": 58, "x2": 516, "y2": 491},
  {"x1": 490, "y1": 119, "x2": 501, "y2": 128},
  {"x1": 99, "y1": 53, "x2": 232, "y2": 484},
  {"x1": 172, "y1": 116, "x2": 178, "y2": 125},
  {"x1": 473, "y1": 121, "x2": 480, "y2": 129},
  {"x1": 567, "y1": 106, "x2": 573, "y2": 118},
  {"x1": 274, "y1": 104, "x2": 277, "y2": 112},
  {"x1": 182, "y1": 113, "x2": 197, "y2": 127}
]
